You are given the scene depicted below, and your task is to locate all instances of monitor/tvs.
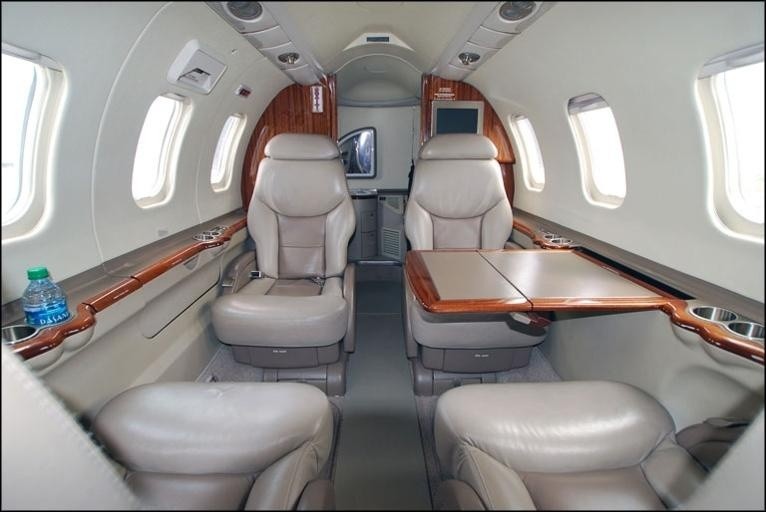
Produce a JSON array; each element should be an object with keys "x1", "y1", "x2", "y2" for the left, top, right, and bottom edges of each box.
[{"x1": 431, "y1": 101, "x2": 484, "y2": 138}]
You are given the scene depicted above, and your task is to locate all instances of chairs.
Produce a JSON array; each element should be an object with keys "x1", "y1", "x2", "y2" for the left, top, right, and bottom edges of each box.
[
  {"x1": 0, "y1": 338, "x2": 336, "y2": 512},
  {"x1": 430, "y1": 381, "x2": 766, "y2": 512},
  {"x1": 211, "y1": 134, "x2": 359, "y2": 368},
  {"x1": 401, "y1": 133, "x2": 549, "y2": 372}
]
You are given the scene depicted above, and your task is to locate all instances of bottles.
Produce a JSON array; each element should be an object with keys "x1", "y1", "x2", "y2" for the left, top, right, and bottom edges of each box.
[{"x1": 20, "y1": 266, "x2": 71, "y2": 328}]
